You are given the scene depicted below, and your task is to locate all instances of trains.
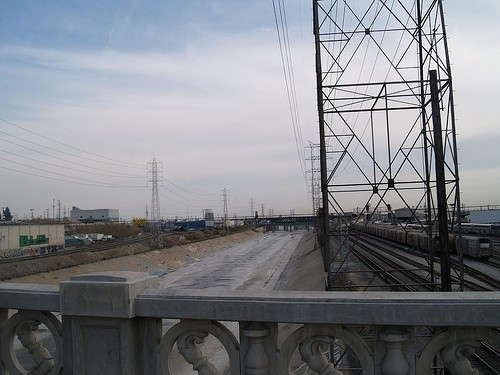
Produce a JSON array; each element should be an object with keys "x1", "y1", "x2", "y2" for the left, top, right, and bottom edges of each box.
[
  {"x1": 379, "y1": 224, "x2": 491, "y2": 263},
  {"x1": 447, "y1": 222, "x2": 500, "y2": 237},
  {"x1": 349, "y1": 219, "x2": 440, "y2": 257}
]
